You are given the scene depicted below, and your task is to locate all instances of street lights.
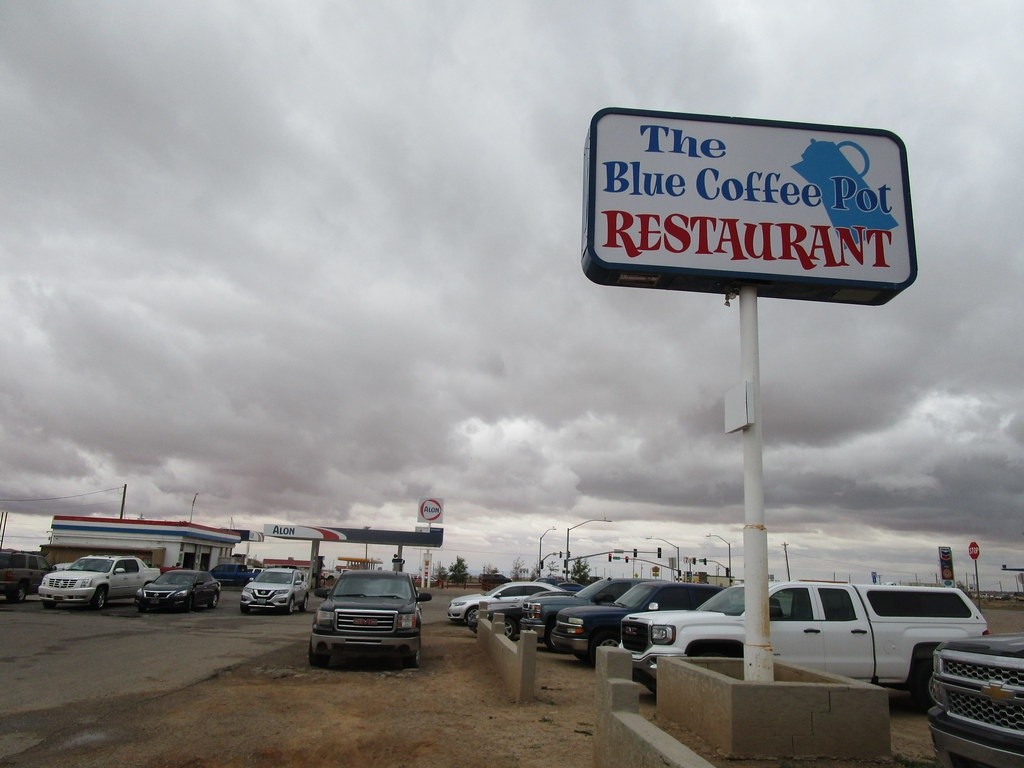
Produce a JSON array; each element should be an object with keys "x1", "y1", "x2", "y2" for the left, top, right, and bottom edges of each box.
[
  {"x1": 646, "y1": 537, "x2": 680, "y2": 582},
  {"x1": 539, "y1": 528, "x2": 557, "y2": 578},
  {"x1": 566, "y1": 518, "x2": 613, "y2": 580}
]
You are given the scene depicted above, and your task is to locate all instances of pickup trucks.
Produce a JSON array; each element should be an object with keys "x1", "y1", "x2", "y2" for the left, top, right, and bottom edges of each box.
[
  {"x1": 38, "y1": 554, "x2": 161, "y2": 610},
  {"x1": 208, "y1": 563, "x2": 259, "y2": 587},
  {"x1": 619, "y1": 580, "x2": 990, "y2": 705}
]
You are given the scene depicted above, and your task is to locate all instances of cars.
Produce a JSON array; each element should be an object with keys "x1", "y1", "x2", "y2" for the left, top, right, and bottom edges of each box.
[
  {"x1": 245, "y1": 568, "x2": 265, "y2": 583},
  {"x1": 447, "y1": 581, "x2": 569, "y2": 628},
  {"x1": 134, "y1": 570, "x2": 221, "y2": 613},
  {"x1": 467, "y1": 591, "x2": 577, "y2": 640},
  {"x1": 925, "y1": 633, "x2": 1024, "y2": 768},
  {"x1": 239, "y1": 568, "x2": 311, "y2": 614},
  {"x1": 551, "y1": 582, "x2": 726, "y2": 665},
  {"x1": 521, "y1": 578, "x2": 677, "y2": 652}
]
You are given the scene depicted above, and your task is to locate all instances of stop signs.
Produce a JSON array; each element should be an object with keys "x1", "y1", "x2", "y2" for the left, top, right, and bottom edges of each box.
[{"x1": 968, "y1": 541, "x2": 979, "y2": 559}]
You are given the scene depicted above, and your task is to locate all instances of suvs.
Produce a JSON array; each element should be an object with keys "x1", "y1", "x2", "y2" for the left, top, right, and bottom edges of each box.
[
  {"x1": 307, "y1": 568, "x2": 433, "y2": 669},
  {"x1": 0, "y1": 551, "x2": 52, "y2": 604}
]
